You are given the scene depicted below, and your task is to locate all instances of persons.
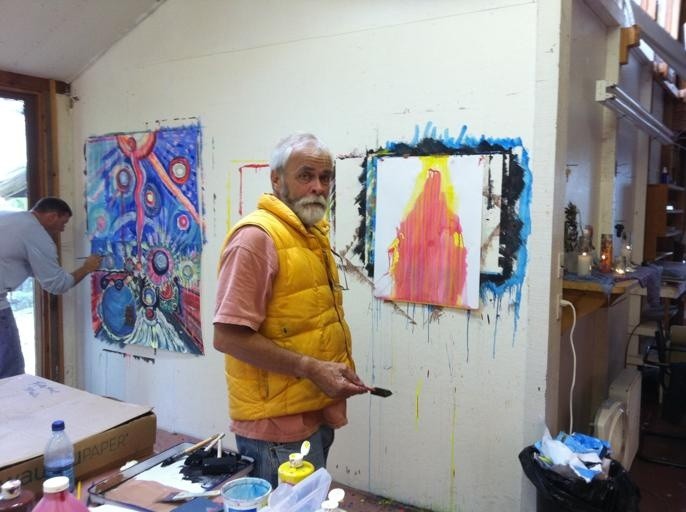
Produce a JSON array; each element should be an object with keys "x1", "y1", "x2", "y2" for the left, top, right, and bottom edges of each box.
[
  {"x1": 0, "y1": 195, "x2": 103, "y2": 379},
  {"x1": 213, "y1": 133, "x2": 368, "y2": 490}
]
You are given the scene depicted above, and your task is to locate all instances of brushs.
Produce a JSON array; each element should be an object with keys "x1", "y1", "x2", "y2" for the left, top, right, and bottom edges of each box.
[{"x1": 156, "y1": 490, "x2": 221, "y2": 503}]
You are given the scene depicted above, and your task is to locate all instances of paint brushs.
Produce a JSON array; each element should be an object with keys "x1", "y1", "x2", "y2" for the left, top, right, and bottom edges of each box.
[
  {"x1": 77, "y1": 253, "x2": 113, "y2": 259},
  {"x1": 184, "y1": 432, "x2": 225, "y2": 466},
  {"x1": 361, "y1": 385, "x2": 392, "y2": 397},
  {"x1": 160, "y1": 432, "x2": 221, "y2": 467}
]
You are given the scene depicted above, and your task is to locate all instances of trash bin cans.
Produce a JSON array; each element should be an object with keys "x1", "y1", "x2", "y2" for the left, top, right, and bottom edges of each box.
[{"x1": 518, "y1": 446, "x2": 626, "y2": 512}]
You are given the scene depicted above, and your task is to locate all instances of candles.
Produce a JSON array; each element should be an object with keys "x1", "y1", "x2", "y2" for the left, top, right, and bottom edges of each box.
[{"x1": 578, "y1": 254, "x2": 592, "y2": 277}]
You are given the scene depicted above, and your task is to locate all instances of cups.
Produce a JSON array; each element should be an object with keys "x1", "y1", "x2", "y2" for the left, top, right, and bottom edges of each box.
[
  {"x1": 220, "y1": 477, "x2": 273, "y2": 512},
  {"x1": 614, "y1": 256, "x2": 627, "y2": 278},
  {"x1": 620, "y1": 243, "x2": 632, "y2": 268}
]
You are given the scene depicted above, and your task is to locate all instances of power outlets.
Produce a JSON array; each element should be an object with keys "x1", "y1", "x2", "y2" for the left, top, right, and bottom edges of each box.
[{"x1": 557, "y1": 294, "x2": 563, "y2": 321}]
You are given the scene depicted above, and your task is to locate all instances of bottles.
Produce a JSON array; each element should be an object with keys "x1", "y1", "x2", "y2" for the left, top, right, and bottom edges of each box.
[
  {"x1": 601, "y1": 233, "x2": 613, "y2": 272},
  {"x1": 276, "y1": 439, "x2": 315, "y2": 487},
  {"x1": 0, "y1": 419, "x2": 92, "y2": 512}
]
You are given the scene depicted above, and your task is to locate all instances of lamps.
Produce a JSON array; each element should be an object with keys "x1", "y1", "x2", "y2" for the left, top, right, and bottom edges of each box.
[{"x1": 593, "y1": 79, "x2": 674, "y2": 148}]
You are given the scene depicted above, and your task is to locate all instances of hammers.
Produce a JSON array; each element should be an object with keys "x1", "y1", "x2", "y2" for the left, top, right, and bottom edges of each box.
[{"x1": 201, "y1": 439, "x2": 237, "y2": 473}]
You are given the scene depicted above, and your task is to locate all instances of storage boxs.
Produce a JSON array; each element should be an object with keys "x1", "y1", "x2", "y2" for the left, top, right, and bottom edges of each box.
[{"x1": 0, "y1": 373, "x2": 156, "y2": 501}]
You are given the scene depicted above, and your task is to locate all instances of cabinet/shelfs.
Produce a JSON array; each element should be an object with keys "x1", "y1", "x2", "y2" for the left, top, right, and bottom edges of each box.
[
  {"x1": 625, "y1": 280, "x2": 685, "y2": 368},
  {"x1": 643, "y1": 182, "x2": 686, "y2": 262}
]
